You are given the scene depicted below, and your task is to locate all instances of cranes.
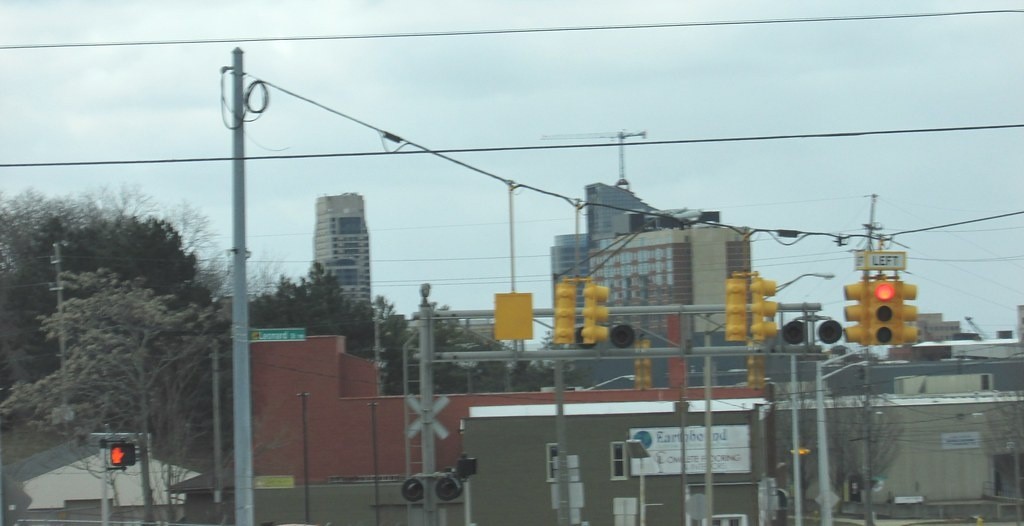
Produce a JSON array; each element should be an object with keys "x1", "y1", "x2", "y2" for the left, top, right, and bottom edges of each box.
[{"x1": 540, "y1": 131, "x2": 647, "y2": 181}]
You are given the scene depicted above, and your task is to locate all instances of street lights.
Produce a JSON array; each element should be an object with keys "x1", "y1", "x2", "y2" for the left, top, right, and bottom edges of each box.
[{"x1": 814, "y1": 347, "x2": 869, "y2": 526}]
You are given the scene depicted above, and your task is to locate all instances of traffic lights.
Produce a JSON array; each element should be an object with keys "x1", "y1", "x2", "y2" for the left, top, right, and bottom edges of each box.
[
  {"x1": 844, "y1": 282, "x2": 868, "y2": 346},
  {"x1": 581, "y1": 282, "x2": 609, "y2": 345},
  {"x1": 750, "y1": 276, "x2": 778, "y2": 340},
  {"x1": 867, "y1": 281, "x2": 897, "y2": 345},
  {"x1": 896, "y1": 280, "x2": 919, "y2": 346},
  {"x1": 458, "y1": 458, "x2": 477, "y2": 478},
  {"x1": 109, "y1": 443, "x2": 136, "y2": 467}
]
